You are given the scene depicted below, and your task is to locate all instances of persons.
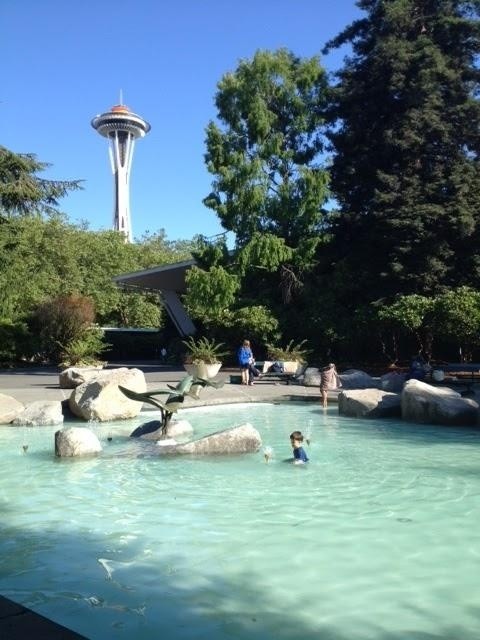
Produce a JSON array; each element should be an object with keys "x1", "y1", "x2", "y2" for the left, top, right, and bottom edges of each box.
[
  {"x1": 238, "y1": 339, "x2": 253, "y2": 385},
  {"x1": 290, "y1": 432, "x2": 310, "y2": 465},
  {"x1": 320, "y1": 363, "x2": 342, "y2": 408},
  {"x1": 249, "y1": 358, "x2": 264, "y2": 386}
]
